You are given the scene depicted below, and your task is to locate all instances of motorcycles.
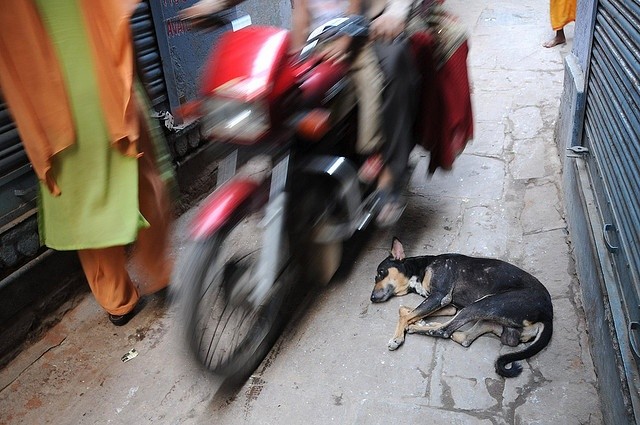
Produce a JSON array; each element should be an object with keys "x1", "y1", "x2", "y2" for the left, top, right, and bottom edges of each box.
[{"x1": 170, "y1": 15, "x2": 474, "y2": 373}]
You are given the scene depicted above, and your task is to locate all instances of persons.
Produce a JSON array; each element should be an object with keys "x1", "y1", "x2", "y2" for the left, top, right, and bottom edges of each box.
[
  {"x1": 541, "y1": 0, "x2": 576, "y2": 47},
  {"x1": 2, "y1": 1, "x2": 172, "y2": 326},
  {"x1": 289, "y1": 1, "x2": 385, "y2": 184},
  {"x1": 405, "y1": 0, "x2": 475, "y2": 182},
  {"x1": 171, "y1": 0, "x2": 418, "y2": 228}
]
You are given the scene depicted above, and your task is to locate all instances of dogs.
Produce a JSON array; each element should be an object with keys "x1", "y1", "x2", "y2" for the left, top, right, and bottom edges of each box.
[{"x1": 370, "y1": 236, "x2": 553, "y2": 378}]
